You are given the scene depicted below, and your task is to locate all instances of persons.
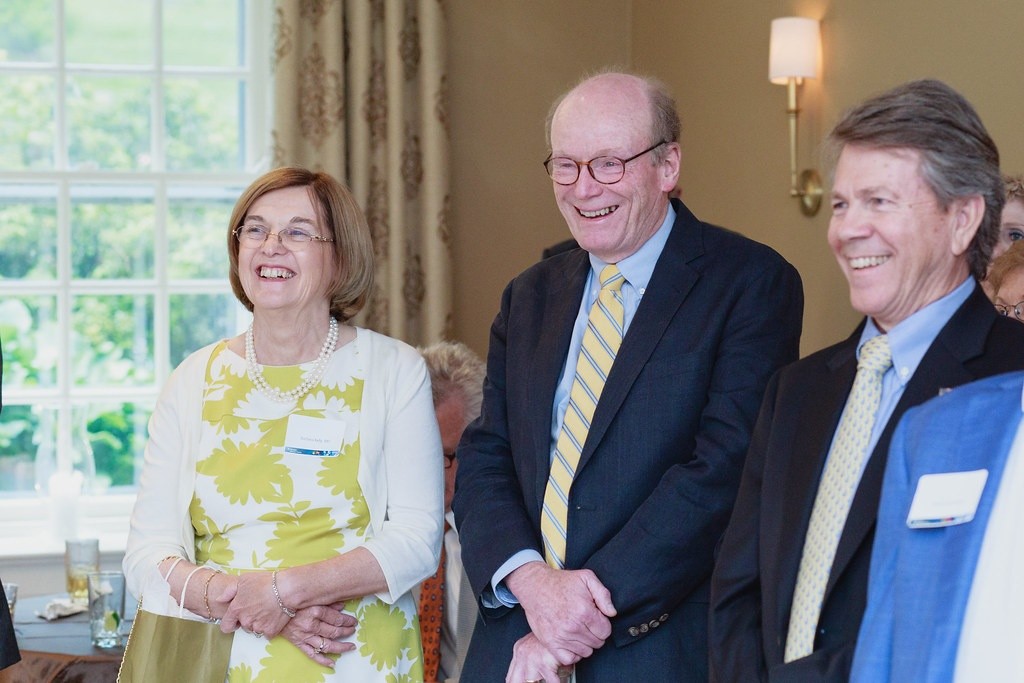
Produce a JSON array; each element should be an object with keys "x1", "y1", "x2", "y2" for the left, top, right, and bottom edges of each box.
[
  {"x1": 707, "y1": 80, "x2": 1024, "y2": 683},
  {"x1": 451, "y1": 72, "x2": 806, "y2": 683},
  {"x1": 974, "y1": 175, "x2": 1024, "y2": 322},
  {"x1": 120, "y1": 166, "x2": 444, "y2": 683},
  {"x1": 847, "y1": 373, "x2": 1024, "y2": 683},
  {"x1": 404, "y1": 343, "x2": 488, "y2": 683}
]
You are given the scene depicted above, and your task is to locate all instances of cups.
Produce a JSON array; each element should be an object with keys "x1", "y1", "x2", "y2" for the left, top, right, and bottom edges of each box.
[
  {"x1": 65, "y1": 539, "x2": 99, "y2": 605},
  {"x1": 3, "y1": 585, "x2": 18, "y2": 623},
  {"x1": 88, "y1": 570, "x2": 125, "y2": 648}
]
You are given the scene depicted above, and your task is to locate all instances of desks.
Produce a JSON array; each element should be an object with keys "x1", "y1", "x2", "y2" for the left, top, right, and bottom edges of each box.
[{"x1": 0, "y1": 585, "x2": 139, "y2": 683}]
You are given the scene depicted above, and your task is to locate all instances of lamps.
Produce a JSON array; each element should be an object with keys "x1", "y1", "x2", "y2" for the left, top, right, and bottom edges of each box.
[{"x1": 768, "y1": 15, "x2": 827, "y2": 217}]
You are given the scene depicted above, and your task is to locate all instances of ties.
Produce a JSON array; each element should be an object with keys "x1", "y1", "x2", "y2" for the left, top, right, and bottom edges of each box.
[
  {"x1": 783, "y1": 334, "x2": 892, "y2": 664},
  {"x1": 418, "y1": 519, "x2": 450, "y2": 683},
  {"x1": 540, "y1": 264, "x2": 624, "y2": 572}
]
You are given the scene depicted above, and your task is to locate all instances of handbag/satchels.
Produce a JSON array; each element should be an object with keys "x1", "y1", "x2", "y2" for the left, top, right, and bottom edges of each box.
[{"x1": 118, "y1": 558, "x2": 235, "y2": 683}]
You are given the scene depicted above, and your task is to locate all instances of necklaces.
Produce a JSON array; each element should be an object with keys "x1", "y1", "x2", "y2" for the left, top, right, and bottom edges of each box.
[{"x1": 247, "y1": 313, "x2": 338, "y2": 404}]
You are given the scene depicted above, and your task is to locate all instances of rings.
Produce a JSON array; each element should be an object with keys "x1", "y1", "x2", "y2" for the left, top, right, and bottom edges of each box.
[
  {"x1": 314, "y1": 638, "x2": 324, "y2": 654},
  {"x1": 253, "y1": 631, "x2": 263, "y2": 637}
]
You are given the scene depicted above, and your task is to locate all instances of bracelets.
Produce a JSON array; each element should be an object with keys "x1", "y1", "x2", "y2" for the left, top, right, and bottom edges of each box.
[
  {"x1": 272, "y1": 569, "x2": 296, "y2": 618},
  {"x1": 204, "y1": 571, "x2": 225, "y2": 623}
]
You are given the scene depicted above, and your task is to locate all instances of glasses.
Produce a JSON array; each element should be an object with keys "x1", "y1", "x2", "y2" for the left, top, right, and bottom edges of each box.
[
  {"x1": 993, "y1": 301, "x2": 1024, "y2": 321},
  {"x1": 232, "y1": 225, "x2": 337, "y2": 251},
  {"x1": 544, "y1": 139, "x2": 673, "y2": 184},
  {"x1": 444, "y1": 451, "x2": 457, "y2": 468}
]
